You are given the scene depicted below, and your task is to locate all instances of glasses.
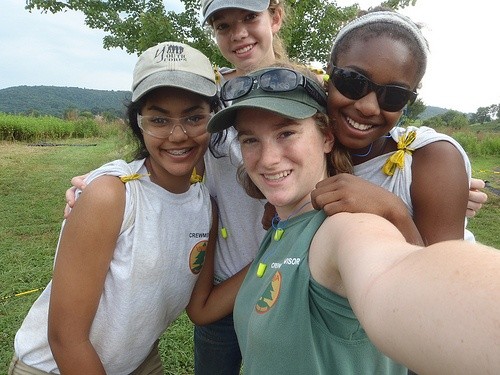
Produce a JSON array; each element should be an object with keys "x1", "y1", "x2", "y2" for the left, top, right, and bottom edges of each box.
[
  {"x1": 330, "y1": 60, "x2": 418, "y2": 113},
  {"x1": 219, "y1": 68, "x2": 326, "y2": 108},
  {"x1": 136, "y1": 112, "x2": 216, "y2": 138}
]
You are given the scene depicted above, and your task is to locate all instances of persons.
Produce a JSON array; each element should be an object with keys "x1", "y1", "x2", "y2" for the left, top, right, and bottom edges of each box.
[
  {"x1": 263, "y1": 8, "x2": 473, "y2": 249},
  {"x1": 64, "y1": 0, "x2": 488, "y2": 375},
  {"x1": 8, "y1": 38, "x2": 280, "y2": 375},
  {"x1": 217, "y1": 59, "x2": 500, "y2": 375}
]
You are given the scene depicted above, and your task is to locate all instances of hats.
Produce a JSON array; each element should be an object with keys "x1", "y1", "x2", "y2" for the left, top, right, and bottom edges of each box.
[
  {"x1": 131, "y1": 41, "x2": 216, "y2": 103},
  {"x1": 205, "y1": 66, "x2": 328, "y2": 133},
  {"x1": 202, "y1": 0, "x2": 272, "y2": 26}
]
